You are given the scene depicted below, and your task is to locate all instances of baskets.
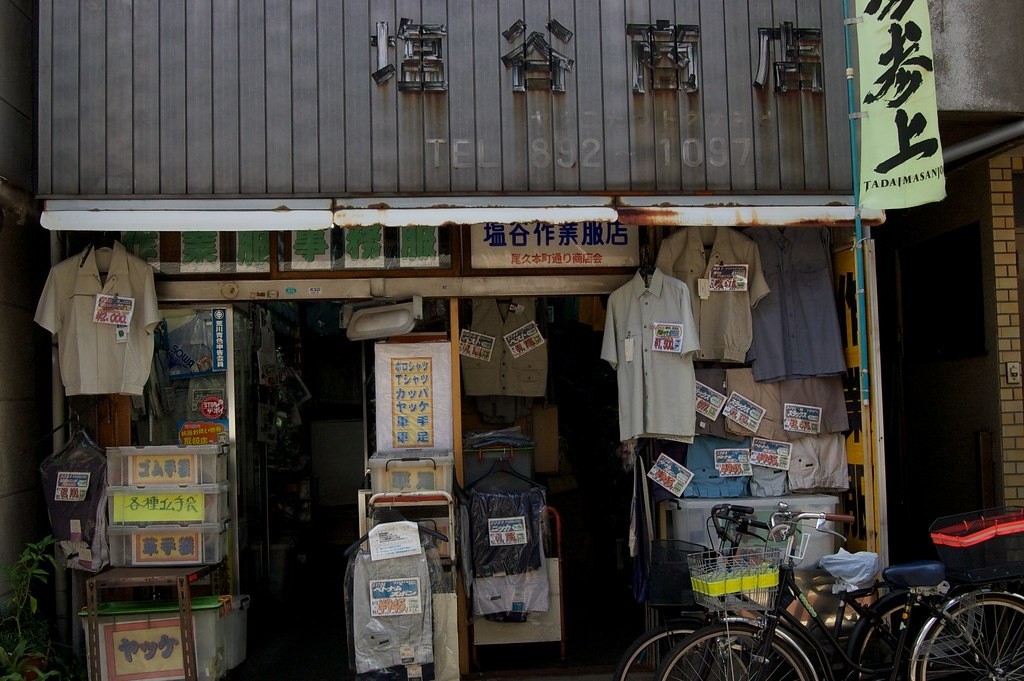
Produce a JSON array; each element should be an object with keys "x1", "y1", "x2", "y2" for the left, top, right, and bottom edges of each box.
[
  {"x1": 687, "y1": 544, "x2": 782, "y2": 611},
  {"x1": 929, "y1": 506, "x2": 1024, "y2": 581},
  {"x1": 648, "y1": 540, "x2": 708, "y2": 606}
]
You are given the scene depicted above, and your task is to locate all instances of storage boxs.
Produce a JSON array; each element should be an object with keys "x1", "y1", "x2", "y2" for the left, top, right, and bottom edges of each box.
[
  {"x1": 367, "y1": 447, "x2": 454, "y2": 497},
  {"x1": 78, "y1": 595, "x2": 232, "y2": 680},
  {"x1": 462, "y1": 404, "x2": 559, "y2": 475},
  {"x1": 367, "y1": 516, "x2": 450, "y2": 558},
  {"x1": 225, "y1": 595, "x2": 251, "y2": 669},
  {"x1": 107, "y1": 518, "x2": 232, "y2": 568},
  {"x1": 669, "y1": 493, "x2": 840, "y2": 576},
  {"x1": 462, "y1": 446, "x2": 536, "y2": 493},
  {"x1": 106, "y1": 444, "x2": 228, "y2": 486},
  {"x1": 106, "y1": 485, "x2": 229, "y2": 524}
]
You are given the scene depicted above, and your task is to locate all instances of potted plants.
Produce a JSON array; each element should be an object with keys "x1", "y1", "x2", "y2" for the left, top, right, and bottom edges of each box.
[{"x1": 0, "y1": 533, "x2": 61, "y2": 681}]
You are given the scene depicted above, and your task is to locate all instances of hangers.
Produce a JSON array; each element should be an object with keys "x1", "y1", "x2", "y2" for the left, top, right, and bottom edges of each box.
[
  {"x1": 639, "y1": 244, "x2": 656, "y2": 278},
  {"x1": 93, "y1": 231, "x2": 114, "y2": 250},
  {"x1": 461, "y1": 445, "x2": 550, "y2": 493},
  {"x1": 39, "y1": 409, "x2": 107, "y2": 467},
  {"x1": 343, "y1": 491, "x2": 450, "y2": 560}
]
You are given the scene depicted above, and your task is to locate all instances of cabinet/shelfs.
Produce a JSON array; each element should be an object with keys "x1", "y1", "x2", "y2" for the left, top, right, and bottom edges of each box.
[
  {"x1": 86, "y1": 555, "x2": 228, "y2": 681},
  {"x1": 358, "y1": 489, "x2": 457, "y2": 596}
]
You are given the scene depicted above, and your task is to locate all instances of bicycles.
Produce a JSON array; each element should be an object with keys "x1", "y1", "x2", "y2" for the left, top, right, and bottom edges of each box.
[
  {"x1": 655, "y1": 501, "x2": 1024, "y2": 681},
  {"x1": 612, "y1": 503, "x2": 980, "y2": 680}
]
